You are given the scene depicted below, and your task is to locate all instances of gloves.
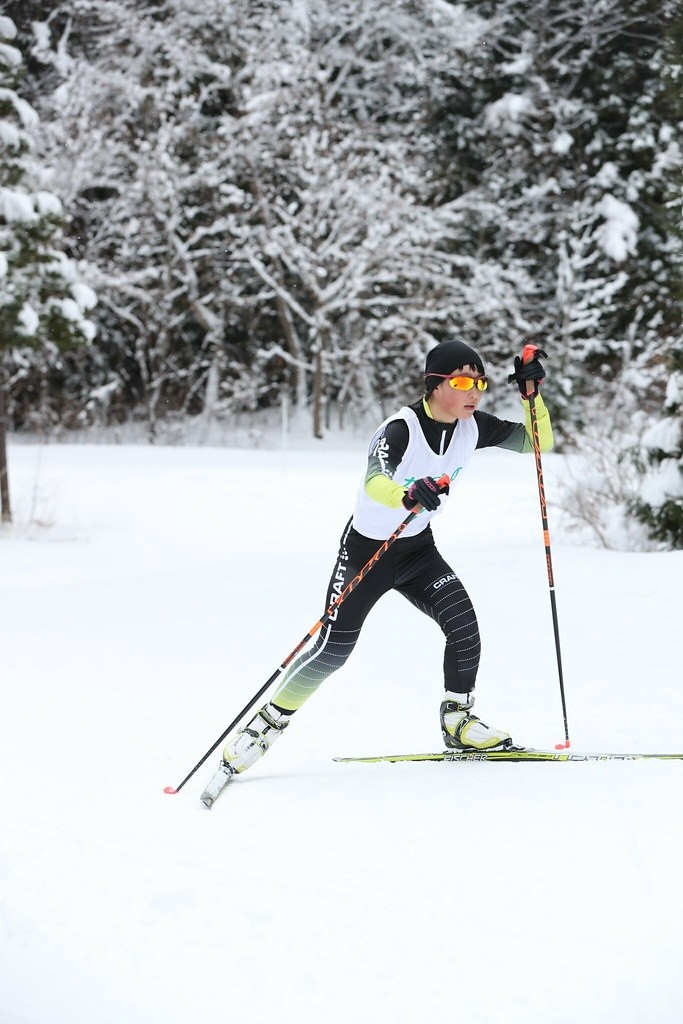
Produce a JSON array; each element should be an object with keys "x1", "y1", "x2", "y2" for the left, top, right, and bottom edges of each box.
[
  {"x1": 508, "y1": 354, "x2": 546, "y2": 400},
  {"x1": 402, "y1": 477, "x2": 449, "y2": 512}
]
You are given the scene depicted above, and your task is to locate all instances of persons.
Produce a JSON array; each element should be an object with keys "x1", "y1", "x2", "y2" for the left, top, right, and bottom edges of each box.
[{"x1": 222, "y1": 339, "x2": 554, "y2": 775}]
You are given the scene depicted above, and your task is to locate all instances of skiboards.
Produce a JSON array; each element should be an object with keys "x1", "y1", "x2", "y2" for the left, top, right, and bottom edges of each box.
[
  {"x1": 200, "y1": 765, "x2": 234, "y2": 810},
  {"x1": 330, "y1": 754, "x2": 683, "y2": 764}
]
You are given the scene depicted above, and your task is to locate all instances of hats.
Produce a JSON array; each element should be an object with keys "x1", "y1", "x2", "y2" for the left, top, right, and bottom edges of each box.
[{"x1": 424, "y1": 339, "x2": 485, "y2": 395}]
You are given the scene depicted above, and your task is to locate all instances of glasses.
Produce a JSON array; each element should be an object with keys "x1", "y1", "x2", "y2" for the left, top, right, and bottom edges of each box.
[{"x1": 449, "y1": 376, "x2": 488, "y2": 392}]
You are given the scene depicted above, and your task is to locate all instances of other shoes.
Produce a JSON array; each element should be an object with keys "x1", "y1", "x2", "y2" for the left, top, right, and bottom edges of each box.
[
  {"x1": 439, "y1": 690, "x2": 513, "y2": 752},
  {"x1": 223, "y1": 703, "x2": 291, "y2": 774}
]
